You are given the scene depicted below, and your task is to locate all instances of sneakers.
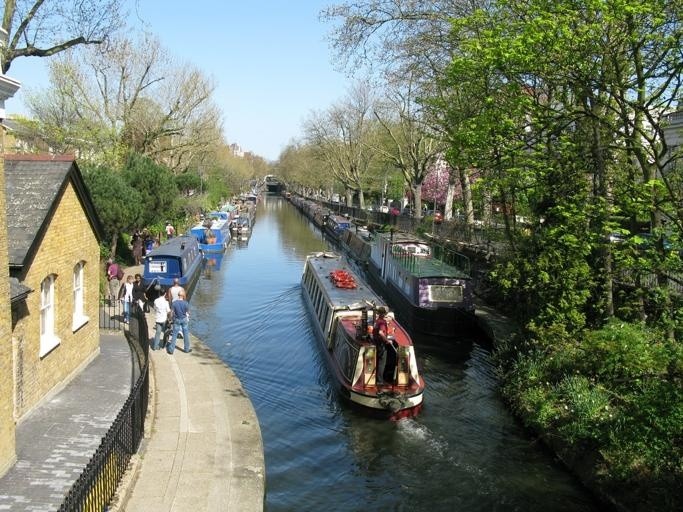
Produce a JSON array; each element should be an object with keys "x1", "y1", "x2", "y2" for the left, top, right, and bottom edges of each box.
[
  {"x1": 153, "y1": 346, "x2": 193, "y2": 354},
  {"x1": 123, "y1": 318, "x2": 129, "y2": 324}
]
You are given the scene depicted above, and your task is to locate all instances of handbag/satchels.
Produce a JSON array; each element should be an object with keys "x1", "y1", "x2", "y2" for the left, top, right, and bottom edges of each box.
[{"x1": 117, "y1": 269, "x2": 124, "y2": 281}]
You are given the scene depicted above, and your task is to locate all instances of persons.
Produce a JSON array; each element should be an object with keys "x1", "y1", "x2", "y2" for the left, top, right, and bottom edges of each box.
[
  {"x1": 108, "y1": 223, "x2": 192, "y2": 354},
  {"x1": 372, "y1": 307, "x2": 393, "y2": 386}
]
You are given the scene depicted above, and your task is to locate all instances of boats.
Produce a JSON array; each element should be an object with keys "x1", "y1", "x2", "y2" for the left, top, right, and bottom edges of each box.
[
  {"x1": 280, "y1": 187, "x2": 372, "y2": 263},
  {"x1": 369, "y1": 228, "x2": 488, "y2": 343},
  {"x1": 300, "y1": 248, "x2": 425, "y2": 423}
]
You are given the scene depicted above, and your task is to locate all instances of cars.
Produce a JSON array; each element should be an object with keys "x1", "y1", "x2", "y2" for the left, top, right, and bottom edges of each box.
[{"x1": 426, "y1": 210, "x2": 443, "y2": 225}]
